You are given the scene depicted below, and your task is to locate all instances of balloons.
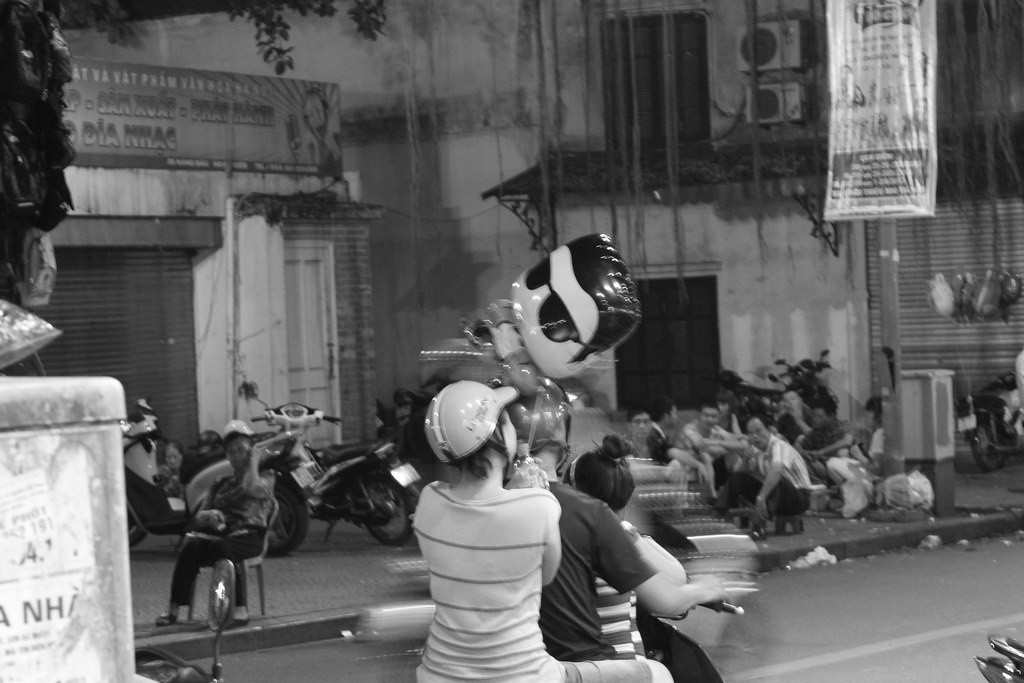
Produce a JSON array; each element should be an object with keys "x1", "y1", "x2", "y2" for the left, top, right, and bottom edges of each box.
[{"x1": 463, "y1": 229, "x2": 644, "y2": 387}]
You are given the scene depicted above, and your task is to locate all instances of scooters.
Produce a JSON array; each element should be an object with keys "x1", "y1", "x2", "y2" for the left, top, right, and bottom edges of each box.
[
  {"x1": 956, "y1": 370, "x2": 1024, "y2": 474},
  {"x1": 718, "y1": 348, "x2": 839, "y2": 444},
  {"x1": 240, "y1": 375, "x2": 476, "y2": 548},
  {"x1": 120, "y1": 396, "x2": 324, "y2": 558}
]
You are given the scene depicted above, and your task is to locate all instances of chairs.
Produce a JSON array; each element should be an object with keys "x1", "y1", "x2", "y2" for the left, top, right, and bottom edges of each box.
[{"x1": 188, "y1": 493, "x2": 279, "y2": 621}]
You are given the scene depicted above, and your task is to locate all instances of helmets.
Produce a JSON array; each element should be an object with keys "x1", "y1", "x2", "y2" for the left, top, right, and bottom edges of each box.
[
  {"x1": 496, "y1": 376, "x2": 570, "y2": 473},
  {"x1": 224, "y1": 419, "x2": 254, "y2": 438},
  {"x1": 424, "y1": 380, "x2": 520, "y2": 463},
  {"x1": 196, "y1": 430, "x2": 221, "y2": 454}
]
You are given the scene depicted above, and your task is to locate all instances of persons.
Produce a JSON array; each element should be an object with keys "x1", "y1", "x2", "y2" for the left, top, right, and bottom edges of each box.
[
  {"x1": 156, "y1": 439, "x2": 186, "y2": 500},
  {"x1": 502, "y1": 375, "x2": 727, "y2": 663},
  {"x1": 412, "y1": 381, "x2": 676, "y2": 683},
  {"x1": 620, "y1": 386, "x2": 812, "y2": 540},
  {"x1": 772, "y1": 379, "x2": 887, "y2": 501},
  {"x1": 560, "y1": 432, "x2": 686, "y2": 659},
  {"x1": 153, "y1": 430, "x2": 278, "y2": 628}
]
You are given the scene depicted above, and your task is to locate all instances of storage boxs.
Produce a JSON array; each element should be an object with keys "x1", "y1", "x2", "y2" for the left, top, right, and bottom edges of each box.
[{"x1": 0, "y1": 372, "x2": 135, "y2": 682}]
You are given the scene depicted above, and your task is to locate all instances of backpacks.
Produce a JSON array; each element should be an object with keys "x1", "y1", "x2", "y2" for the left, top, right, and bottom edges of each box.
[{"x1": 0, "y1": 0, "x2": 75, "y2": 308}]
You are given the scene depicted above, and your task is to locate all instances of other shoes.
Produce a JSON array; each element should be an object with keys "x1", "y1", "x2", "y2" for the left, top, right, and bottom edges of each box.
[
  {"x1": 223, "y1": 613, "x2": 250, "y2": 629},
  {"x1": 156, "y1": 614, "x2": 191, "y2": 626},
  {"x1": 751, "y1": 523, "x2": 765, "y2": 540}
]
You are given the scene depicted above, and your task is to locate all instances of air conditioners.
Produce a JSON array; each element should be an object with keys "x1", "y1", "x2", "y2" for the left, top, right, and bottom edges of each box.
[
  {"x1": 736, "y1": 17, "x2": 818, "y2": 77},
  {"x1": 745, "y1": 82, "x2": 807, "y2": 125}
]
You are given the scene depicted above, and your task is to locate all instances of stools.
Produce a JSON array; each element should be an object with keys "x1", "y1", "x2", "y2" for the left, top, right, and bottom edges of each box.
[
  {"x1": 729, "y1": 507, "x2": 758, "y2": 530},
  {"x1": 775, "y1": 514, "x2": 806, "y2": 536}
]
